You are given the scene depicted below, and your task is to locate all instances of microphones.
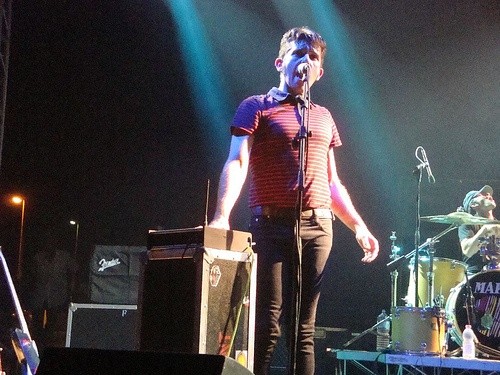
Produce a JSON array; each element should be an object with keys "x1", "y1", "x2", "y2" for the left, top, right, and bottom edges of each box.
[
  {"x1": 297, "y1": 63, "x2": 311, "y2": 74},
  {"x1": 420, "y1": 149, "x2": 435, "y2": 185},
  {"x1": 465, "y1": 291, "x2": 476, "y2": 327}
]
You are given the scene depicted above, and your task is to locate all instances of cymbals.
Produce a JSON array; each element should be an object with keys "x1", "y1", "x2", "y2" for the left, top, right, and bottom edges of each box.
[{"x1": 417, "y1": 212, "x2": 500, "y2": 226}]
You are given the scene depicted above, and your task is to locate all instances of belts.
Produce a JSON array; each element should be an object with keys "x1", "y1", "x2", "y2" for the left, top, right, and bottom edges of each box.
[{"x1": 250, "y1": 207, "x2": 332, "y2": 219}]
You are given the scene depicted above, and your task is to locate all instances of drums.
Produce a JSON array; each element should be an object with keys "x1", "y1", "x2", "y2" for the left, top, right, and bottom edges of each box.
[
  {"x1": 445, "y1": 268, "x2": 500, "y2": 359},
  {"x1": 405, "y1": 256, "x2": 468, "y2": 312},
  {"x1": 391, "y1": 307, "x2": 445, "y2": 356}
]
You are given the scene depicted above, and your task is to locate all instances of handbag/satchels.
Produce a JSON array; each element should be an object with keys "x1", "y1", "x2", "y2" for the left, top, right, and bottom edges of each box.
[{"x1": 8, "y1": 327, "x2": 40, "y2": 375}]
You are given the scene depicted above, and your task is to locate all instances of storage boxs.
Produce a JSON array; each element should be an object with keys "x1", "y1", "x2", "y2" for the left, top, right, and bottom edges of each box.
[
  {"x1": 66, "y1": 303, "x2": 137, "y2": 350},
  {"x1": 136, "y1": 249, "x2": 258, "y2": 370}
]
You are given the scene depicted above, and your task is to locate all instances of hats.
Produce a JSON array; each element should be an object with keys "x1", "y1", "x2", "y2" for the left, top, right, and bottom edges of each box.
[{"x1": 463, "y1": 185, "x2": 493, "y2": 214}]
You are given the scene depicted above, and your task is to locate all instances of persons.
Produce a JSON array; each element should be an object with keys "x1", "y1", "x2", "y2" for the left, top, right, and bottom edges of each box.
[
  {"x1": 207, "y1": 27, "x2": 380, "y2": 375},
  {"x1": 457, "y1": 185, "x2": 500, "y2": 274}
]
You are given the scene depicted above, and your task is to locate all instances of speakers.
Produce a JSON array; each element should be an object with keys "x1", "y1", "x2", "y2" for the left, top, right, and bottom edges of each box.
[{"x1": 33, "y1": 346, "x2": 254, "y2": 375}]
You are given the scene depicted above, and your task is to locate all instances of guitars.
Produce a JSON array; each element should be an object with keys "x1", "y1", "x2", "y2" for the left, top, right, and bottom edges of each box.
[{"x1": 0, "y1": 247, "x2": 40, "y2": 375}]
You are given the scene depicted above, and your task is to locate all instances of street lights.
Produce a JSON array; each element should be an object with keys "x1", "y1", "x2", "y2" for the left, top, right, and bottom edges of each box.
[
  {"x1": 70, "y1": 220, "x2": 79, "y2": 253},
  {"x1": 13, "y1": 196, "x2": 25, "y2": 276}
]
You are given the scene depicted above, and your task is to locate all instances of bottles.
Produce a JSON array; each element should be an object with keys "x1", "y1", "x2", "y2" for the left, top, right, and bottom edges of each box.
[
  {"x1": 463, "y1": 325, "x2": 475, "y2": 359},
  {"x1": 375, "y1": 309, "x2": 390, "y2": 352}
]
